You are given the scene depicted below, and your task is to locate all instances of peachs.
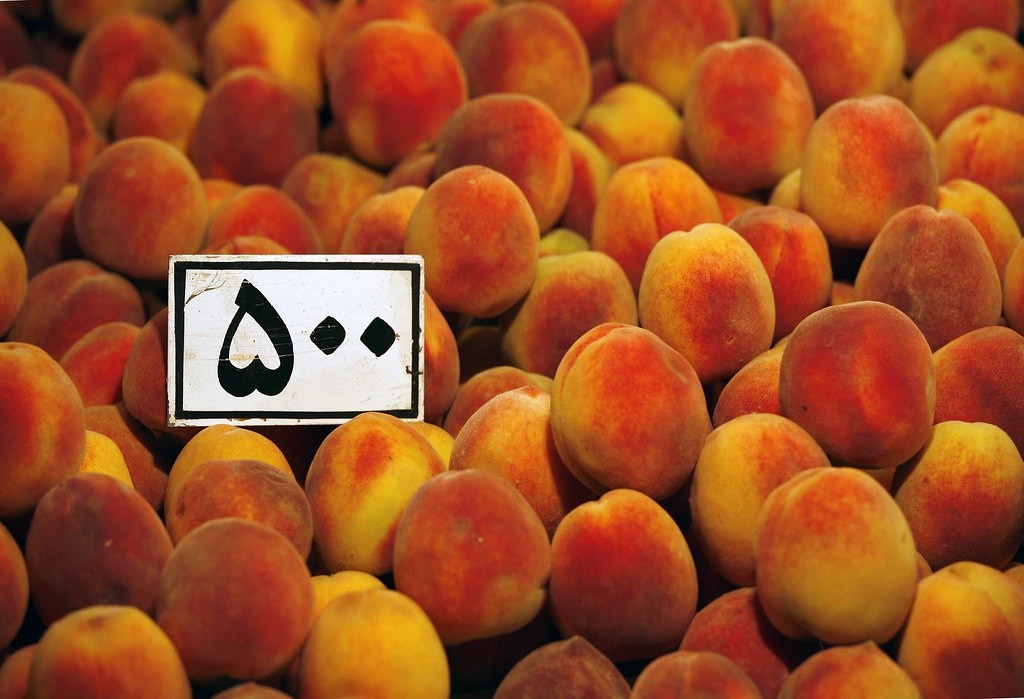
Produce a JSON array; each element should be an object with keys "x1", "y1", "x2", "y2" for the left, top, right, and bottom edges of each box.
[{"x1": 0, "y1": 0, "x2": 1024, "y2": 699}]
